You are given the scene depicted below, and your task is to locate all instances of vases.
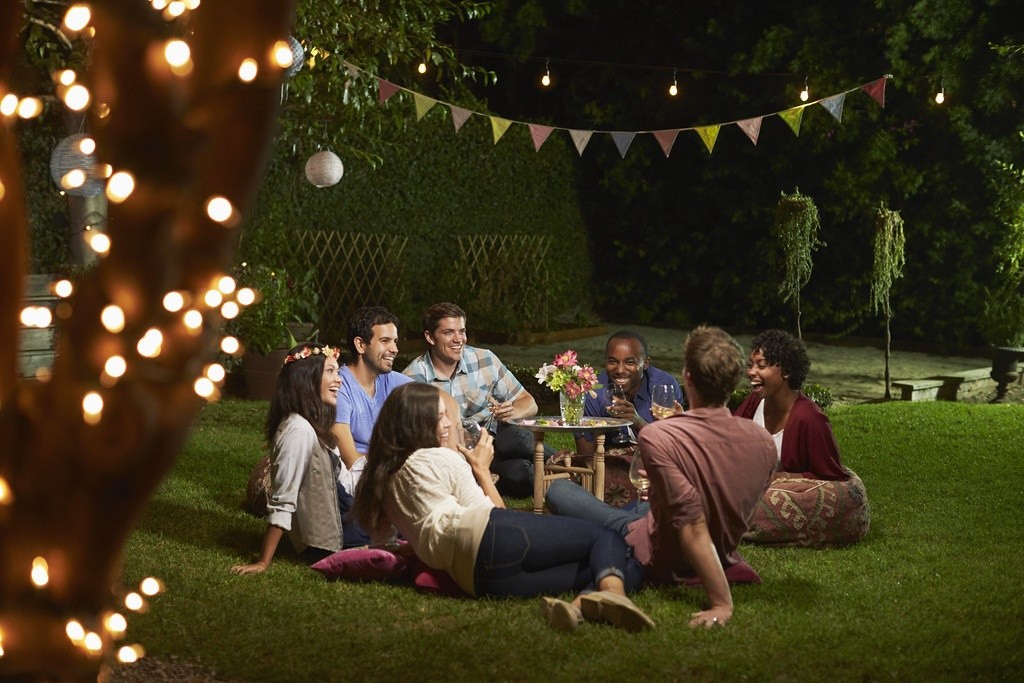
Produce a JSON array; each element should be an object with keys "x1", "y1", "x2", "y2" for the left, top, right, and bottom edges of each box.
[{"x1": 560, "y1": 390, "x2": 585, "y2": 426}]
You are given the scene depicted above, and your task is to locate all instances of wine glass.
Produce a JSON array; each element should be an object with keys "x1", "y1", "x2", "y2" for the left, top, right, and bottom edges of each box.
[
  {"x1": 603, "y1": 384, "x2": 632, "y2": 444},
  {"x1": 455, "y1": 418, "x2": 500, "y2": 486},
  {"x1": 480, "y1": 382, "x2": 510, "y2": 434},
  {"x1": 629, "y1": 449, "x2": 652, "y2": 514}
]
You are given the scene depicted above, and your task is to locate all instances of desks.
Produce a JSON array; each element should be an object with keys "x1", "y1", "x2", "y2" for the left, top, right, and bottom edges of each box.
[{"x1": 507, "y1": 416, "x2": 633, "y2": 515}]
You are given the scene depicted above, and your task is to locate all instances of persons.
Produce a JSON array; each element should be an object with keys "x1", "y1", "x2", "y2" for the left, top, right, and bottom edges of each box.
[
  {"x1": 343, "y1": 382, "x2": 657, "y2": 632},
  {"x1": 572, "y1": 331, "x2": 684, "y2": 468},
  {"x1": 546, "y1": 323, "x2": 778, "y2": 630},
  {"x1": 400, "y1": 302, "x2": 568, "y2": 500},
  {"x1": 230, "y1": 343, "x2": 373, "y2": 576},
  {"x1": 330, "y1": 305, "x2": 416, "y2": 471},
  {"x1": 732, "y1": 328, "x2": 850, "y2": 483}
]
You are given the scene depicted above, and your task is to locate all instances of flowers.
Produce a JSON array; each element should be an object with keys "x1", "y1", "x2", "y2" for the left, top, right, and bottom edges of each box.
[
  {"x1": 283, "y1": 343, "x2": 340, "y2": 367},
  {"x1": 534, "y1": 349, "x2": 604, "y2": 400}
]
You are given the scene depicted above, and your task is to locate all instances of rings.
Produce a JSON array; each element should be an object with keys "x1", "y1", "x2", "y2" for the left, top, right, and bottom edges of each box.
[{"x1": 713, "y1": 617, "x2": 717, "y2": 623}]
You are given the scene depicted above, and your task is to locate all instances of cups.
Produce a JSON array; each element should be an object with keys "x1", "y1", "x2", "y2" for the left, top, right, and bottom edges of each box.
[{"x1": 651, "y1": 385, "x2": 676, "y2": 420}]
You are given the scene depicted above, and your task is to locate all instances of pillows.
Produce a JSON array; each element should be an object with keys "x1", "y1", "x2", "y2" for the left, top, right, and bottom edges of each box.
[{"x1": 311, "y1": 539, "x2": 419, "y2": 580}]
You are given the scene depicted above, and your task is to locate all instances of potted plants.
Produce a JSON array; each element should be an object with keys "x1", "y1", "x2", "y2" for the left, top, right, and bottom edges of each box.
[
  {"x1": 233, "y1": 264, "x2": 320, "y2": 401},
  {"x1": 983, "y1": 280, "x2": 1024, "y2": 404}
]
